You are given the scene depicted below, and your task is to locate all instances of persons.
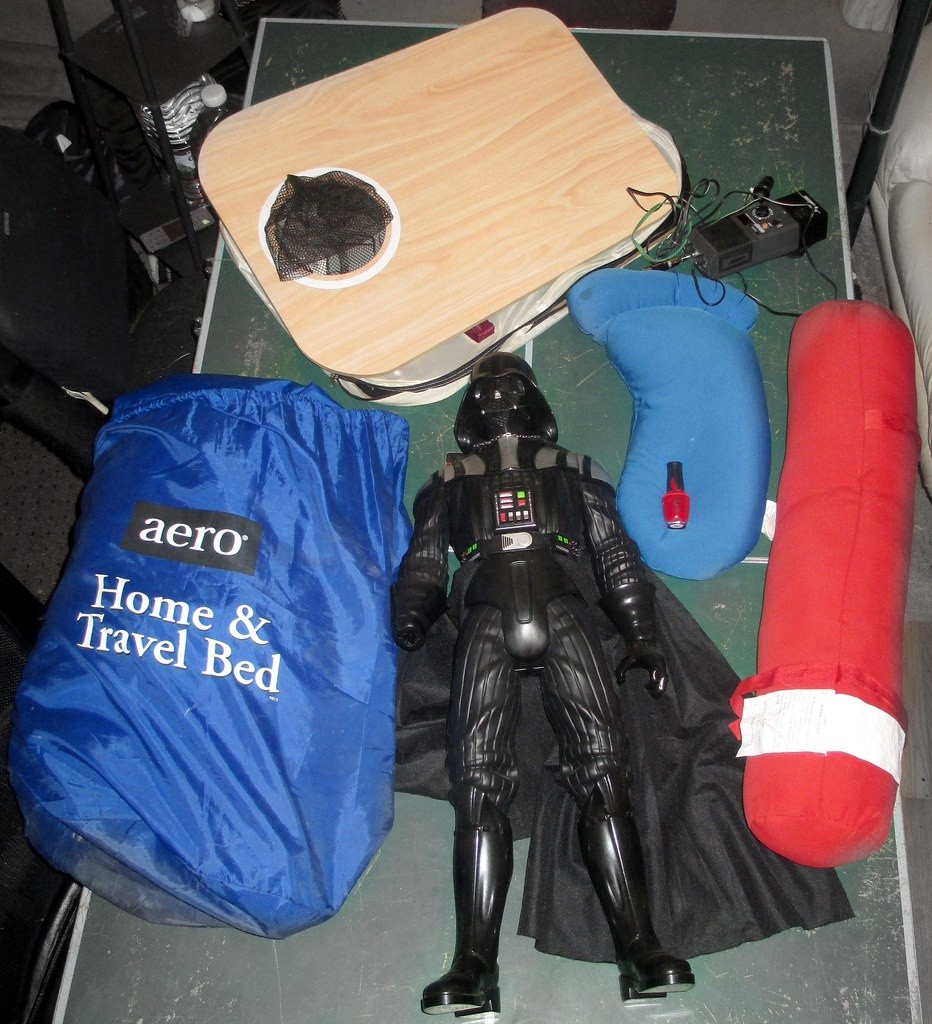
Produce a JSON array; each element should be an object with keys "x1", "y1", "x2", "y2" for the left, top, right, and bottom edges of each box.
[{"x1": 387, "y1": 349, "x2": 699, "y2": 1024}]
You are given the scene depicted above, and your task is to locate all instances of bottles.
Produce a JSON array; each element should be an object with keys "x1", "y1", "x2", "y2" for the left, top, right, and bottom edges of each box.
[
  {"x1": 188, "y1": 83, "x2": 241, "y2": 164},
  {"x1": 662, "y1": 461, "x2": 690, "y2": 530},
  {"x1": 126, "y1": 73, "x2": 219, "y2": 208}
]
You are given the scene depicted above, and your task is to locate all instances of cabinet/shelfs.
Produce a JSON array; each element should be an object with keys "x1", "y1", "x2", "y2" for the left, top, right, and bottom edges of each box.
[{"x1": 48, "y1": 0, "x2": 254, "y2": 280}]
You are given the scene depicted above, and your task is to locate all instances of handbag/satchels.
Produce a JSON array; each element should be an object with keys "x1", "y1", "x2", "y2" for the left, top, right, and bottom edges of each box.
[{"x1": 8, "y1": 370, "x2": 413, "y2": 944}]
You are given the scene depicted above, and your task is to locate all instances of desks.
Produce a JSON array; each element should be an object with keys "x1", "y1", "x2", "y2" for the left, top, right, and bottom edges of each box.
[{"x1": 47, "y1": 15, "x2": 923, "y2": 1024}]
